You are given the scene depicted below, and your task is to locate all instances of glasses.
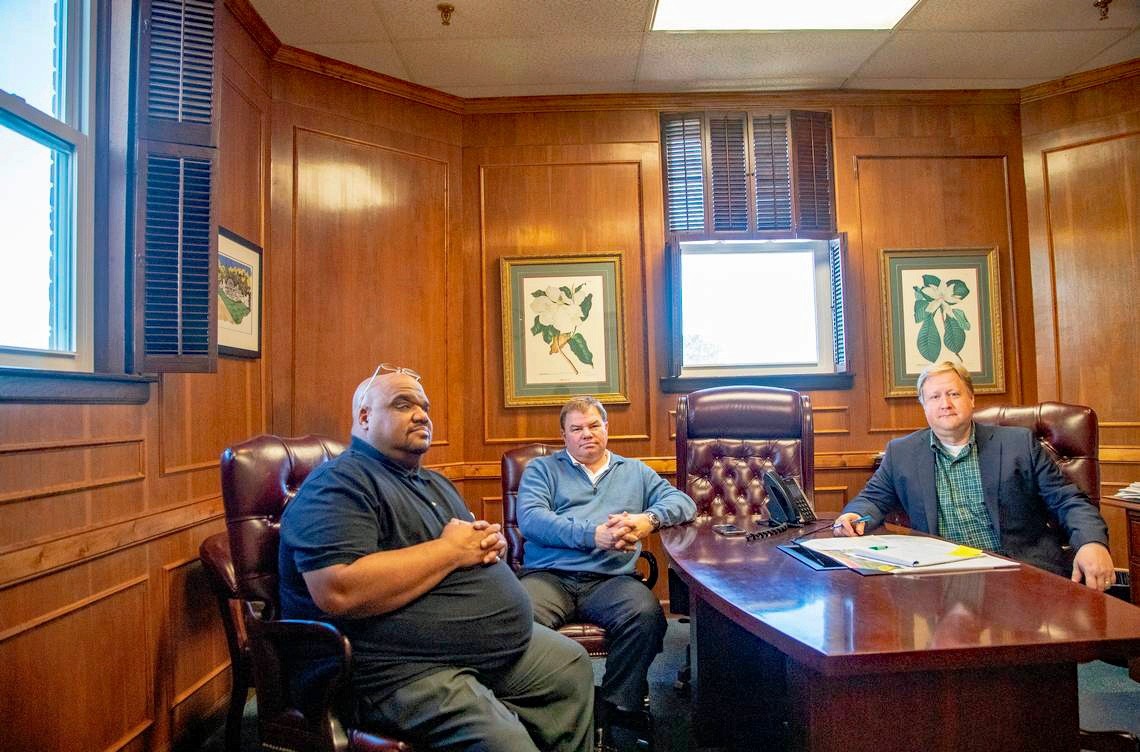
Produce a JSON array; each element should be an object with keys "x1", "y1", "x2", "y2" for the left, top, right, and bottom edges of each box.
[{"x1": 358, "y1": 362, "x2": 421, "y2": 408}]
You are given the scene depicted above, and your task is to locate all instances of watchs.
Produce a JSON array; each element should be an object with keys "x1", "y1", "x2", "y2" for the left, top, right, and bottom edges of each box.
[{"x1": 648, "y1": 512, "x2": 661, "y2": 529}]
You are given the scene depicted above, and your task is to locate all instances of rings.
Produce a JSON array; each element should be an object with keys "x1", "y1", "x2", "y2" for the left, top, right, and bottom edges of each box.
[{"x1": 1104, "y1": 581, "x2": 1111, "y2": 584}]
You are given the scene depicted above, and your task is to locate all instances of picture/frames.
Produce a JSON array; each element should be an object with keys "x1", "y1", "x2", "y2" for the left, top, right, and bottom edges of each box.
[
  {"x1": 500, "y1": 251, "x2": 631, "y2": 408},
  {"x1": 878, "y1": 245, "x2": 1006, "y2": 398},
  {"x1": 217, "y1": 225, "x2": 263, "y2": 359}
]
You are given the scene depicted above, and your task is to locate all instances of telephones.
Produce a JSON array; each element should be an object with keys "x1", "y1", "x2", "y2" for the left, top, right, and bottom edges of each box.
[{"x1": 763, "y1": 470, "x2": 819, "y2": 525}]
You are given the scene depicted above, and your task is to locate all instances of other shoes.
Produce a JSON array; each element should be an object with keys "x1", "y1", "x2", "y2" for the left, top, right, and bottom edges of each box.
[{"x1": 600, "y1": 699, "x2": 655, "y2": 752}]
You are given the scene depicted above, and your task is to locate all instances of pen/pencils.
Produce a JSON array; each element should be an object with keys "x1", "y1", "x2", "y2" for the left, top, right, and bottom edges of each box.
[{"x1": 830, "y1": 516, "x2": 871, "y2": 530}]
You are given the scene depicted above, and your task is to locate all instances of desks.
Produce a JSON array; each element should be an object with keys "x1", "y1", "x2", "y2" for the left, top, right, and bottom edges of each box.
[
  {"x1": 654, "y1": 511, "x2": 1140, "y2": 752},
  {"x1": 1100, "y1": 498, "x2": 1140, "y2": 684}
]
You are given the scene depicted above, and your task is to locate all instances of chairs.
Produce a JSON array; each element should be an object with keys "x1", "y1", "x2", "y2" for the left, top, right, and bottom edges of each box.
[
  {"x1": 198, "y1": 434, "x2": 409, "y2": 752},
  {"x1": 972, "y1": 401, "x2": 1099, "y2": 583},
  {"x1": 669, "y1": 385, "x2": 814, "y2": 688},
  {"x1": 502, "y1": 442, "x2": 659, "y2": 658}
]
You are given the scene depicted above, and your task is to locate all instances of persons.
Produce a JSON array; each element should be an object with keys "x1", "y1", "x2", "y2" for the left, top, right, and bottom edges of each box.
[
  {"x1": 274, "y1": 370, "x2": 597, "y2": 752},
  {"x1": 834, "y1": 362, "x2": 1117, "y2": 597},
  {"x1": 516, "y1": 395, "x2": 697, "y2": 751}
]
